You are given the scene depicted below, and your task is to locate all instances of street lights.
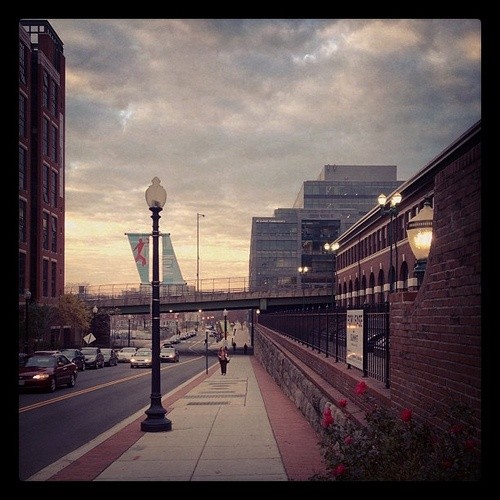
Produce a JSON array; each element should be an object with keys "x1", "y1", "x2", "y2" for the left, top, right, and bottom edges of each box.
[
  {"x1": 322, "y1": 241, "x2": 339, "y2": 308},
  {"x1": 298, "y1": 266, "x2": 309, "y2": 309},
  {"x1": 141, "y1": 175, "x2": 173, "y2": 433},
  {"x1": 377, "y1": 190, "x2": 402, "y2": 293},
  {"x1": 223, "y1": 309, "x2": 229, "y2": 352}
]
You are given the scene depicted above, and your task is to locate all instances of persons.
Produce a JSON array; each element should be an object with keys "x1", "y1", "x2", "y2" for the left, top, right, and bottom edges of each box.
[
  {"x1": 218, "y1": 346, "x2": 228, "y2": 376},
  {"x1": 244, "y1": 343, "x2": 248, "y2": 354},
  {"x1": 233, "y1": 342, "x2": 237, "y2": 351}
]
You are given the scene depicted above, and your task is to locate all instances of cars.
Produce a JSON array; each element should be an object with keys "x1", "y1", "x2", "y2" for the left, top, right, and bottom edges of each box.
[
  {"x1": 160, "y1": 325, "x2": 217, "y2": 348},
  {"x1": 100, "y1": 349, "x2": 118, "y2": 366},
  {"x1": 18, "y1": 353, "x2": 78, "y2": 393},
  {"x1": 59, "y1": 348, "x2": 85, "y2": 372},
  {"x1": 159, "y1": 347, "x2": 180, "y2": 363},
  {"x1": 130, "y1": 347, "x2": 152, "y2": 368},
  {"x1": 28, "y1": 351, "x2": 64, "y2": 365},
  {"x1": 80, "y1": 347, "x2": 105, "y2": 369},
  {"x1": 117, "y1": 347, "x2": 137, "y2": 362}
]
